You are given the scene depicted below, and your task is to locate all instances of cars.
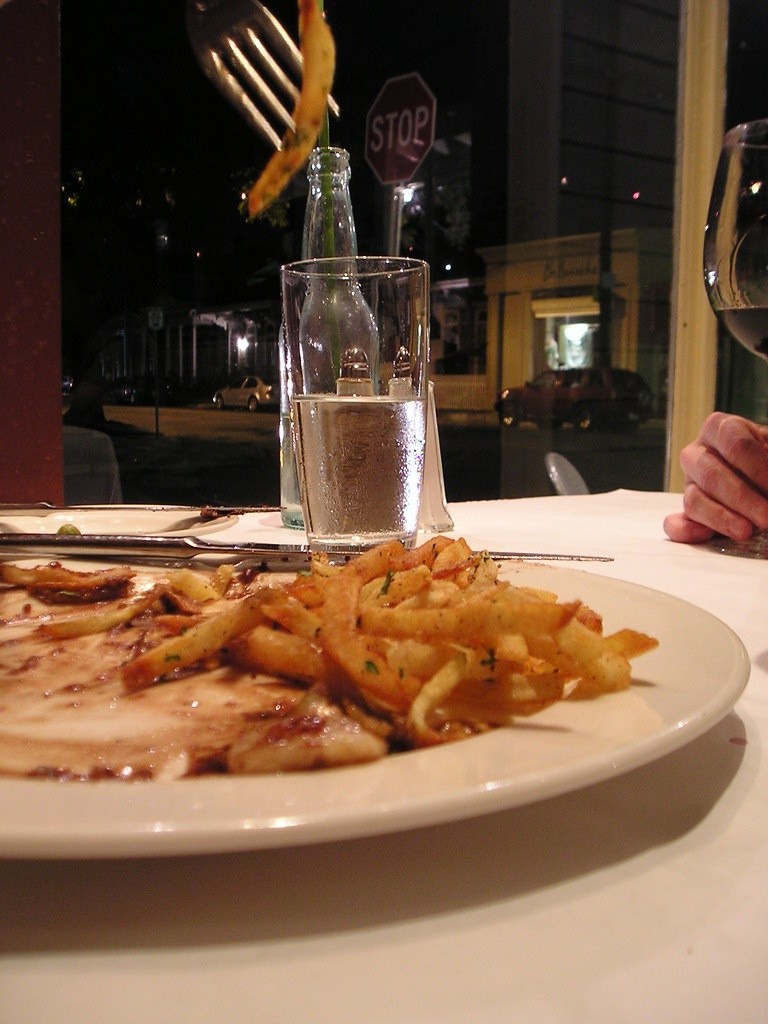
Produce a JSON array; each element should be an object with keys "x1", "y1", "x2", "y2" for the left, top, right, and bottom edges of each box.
[
  {"x1": 103, "y1": 376, "x2": 186, "y2": 406},
  {"x1": 212, "y1": 374, "x2": 280, "y2": 413}
]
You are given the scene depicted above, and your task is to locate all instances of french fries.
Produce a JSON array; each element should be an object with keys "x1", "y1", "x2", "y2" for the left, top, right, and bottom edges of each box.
[
  {"x1": 0, "y1": 536, "x2": 663, "y2": 741},
  {"x1": 245, "y1": 0, "x2": 335, "y2": 218}
]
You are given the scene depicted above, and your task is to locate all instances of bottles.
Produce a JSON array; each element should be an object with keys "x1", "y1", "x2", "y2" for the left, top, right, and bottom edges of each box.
[
  {"x1": 335, "y1": 348, "x2": 373, "y2": 397},
  {"x1": 277, "y1": 147, "x2": 375, "y2": 526},
  {"x1": 388, "y1": 346, "x2": 454, "y2": 533}
]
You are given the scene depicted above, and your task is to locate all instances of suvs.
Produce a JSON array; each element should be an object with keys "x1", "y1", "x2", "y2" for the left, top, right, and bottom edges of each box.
[{"x1": 494, "y1": 366, "x2": 655, "y2": 435}]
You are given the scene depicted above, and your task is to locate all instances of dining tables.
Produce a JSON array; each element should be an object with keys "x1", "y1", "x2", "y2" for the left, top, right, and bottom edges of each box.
[{"x1": 0, "y1": 488, "x2": 767, "y2": 1024}]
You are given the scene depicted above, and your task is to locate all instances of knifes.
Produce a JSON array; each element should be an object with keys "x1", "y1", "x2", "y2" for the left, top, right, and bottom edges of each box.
[
  {"x1": 0, "y1": 501, "x2": 287, "y2": 511},
  {"x1": 0, "y1": 533, "x2": 615, "y2": 564}
]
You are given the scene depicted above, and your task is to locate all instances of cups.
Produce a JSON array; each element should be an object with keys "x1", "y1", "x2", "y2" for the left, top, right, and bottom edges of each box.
[{"x1": 280, "y1": 256, "x2": 431, "y2": 550}]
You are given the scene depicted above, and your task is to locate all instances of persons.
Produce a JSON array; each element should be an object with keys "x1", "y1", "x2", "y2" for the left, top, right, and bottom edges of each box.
[{"x1": 663, "y1": 411, "x2": 768, "y2": 545}]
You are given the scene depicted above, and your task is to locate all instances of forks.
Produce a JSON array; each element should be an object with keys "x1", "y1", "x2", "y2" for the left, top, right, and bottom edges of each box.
[{"x1": 176, "y1": 1, "x2": 340, "y2": 149}]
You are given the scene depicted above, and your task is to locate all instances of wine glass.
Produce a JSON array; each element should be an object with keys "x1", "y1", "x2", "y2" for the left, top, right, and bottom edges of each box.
[{"x1": 703, "y1": 119, "x2": 768, "y2": 560}]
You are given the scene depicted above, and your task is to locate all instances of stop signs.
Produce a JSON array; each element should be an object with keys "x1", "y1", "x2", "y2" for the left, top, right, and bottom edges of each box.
[{"x1": 364, "y1": 71, "x2": 438, "y2": 184}]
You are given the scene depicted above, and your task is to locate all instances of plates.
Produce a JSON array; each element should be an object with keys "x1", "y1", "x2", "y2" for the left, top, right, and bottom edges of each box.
[
  {"x1": 1, "y1": 561, "x2": 750, "y2": 855},
  {"x1": 0, "y1": 503, "x2": 238, "y2": 537},
  {"x1": 194, "y1": 515, "x2": 310, "y2": 546}
]
